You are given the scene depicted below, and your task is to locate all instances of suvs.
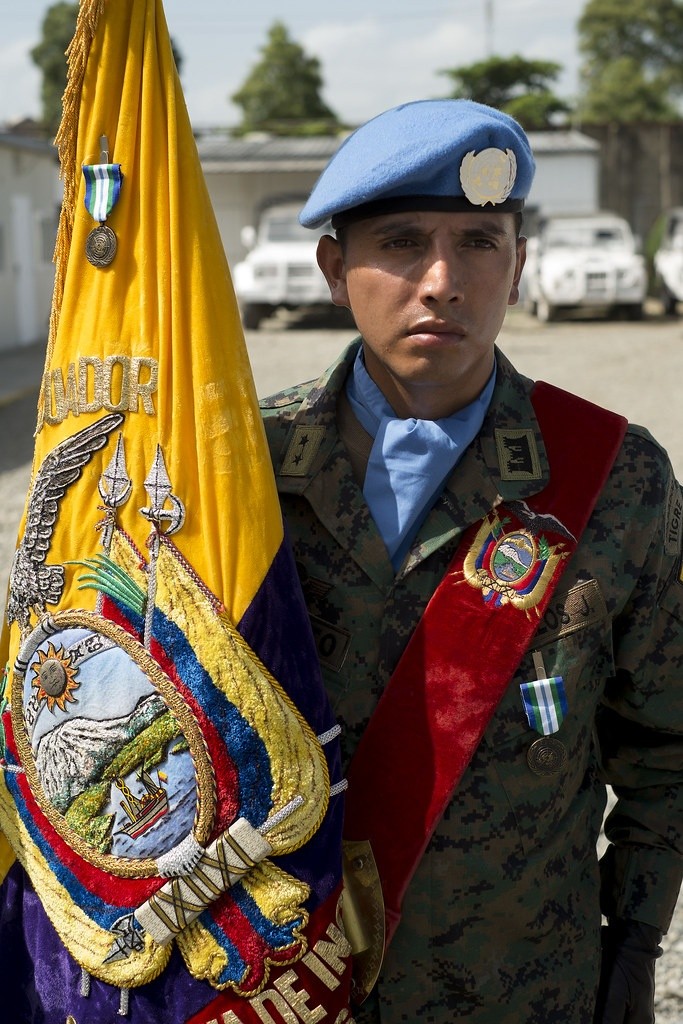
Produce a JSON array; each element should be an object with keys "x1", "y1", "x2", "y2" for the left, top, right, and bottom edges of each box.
[{"x1": 232, "y1": 200, "x2": 347, "y2": 328}]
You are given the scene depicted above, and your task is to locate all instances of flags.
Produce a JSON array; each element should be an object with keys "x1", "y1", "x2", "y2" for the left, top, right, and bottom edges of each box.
[{"x1": 0, "y1": 0, "x2": 353, "y2": 1024}]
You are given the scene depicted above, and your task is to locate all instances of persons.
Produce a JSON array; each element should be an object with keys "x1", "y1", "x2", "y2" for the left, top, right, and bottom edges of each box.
[{"x1": 0, "y1": 98, "x2": 683, "y2": 1024}]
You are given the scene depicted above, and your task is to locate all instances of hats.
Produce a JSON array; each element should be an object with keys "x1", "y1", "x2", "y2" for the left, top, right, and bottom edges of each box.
[{"x1": 299, "y1": 99, "x2": 536, "y2": 230}]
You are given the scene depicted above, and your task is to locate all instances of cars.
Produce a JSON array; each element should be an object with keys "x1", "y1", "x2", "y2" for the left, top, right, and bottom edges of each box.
[
  {"x1": 524, "y1": 215, "x2": 646, "y2": 321},
  {"x1": 654, "y1": 215, "x2": 682, "y2": 315}
]
